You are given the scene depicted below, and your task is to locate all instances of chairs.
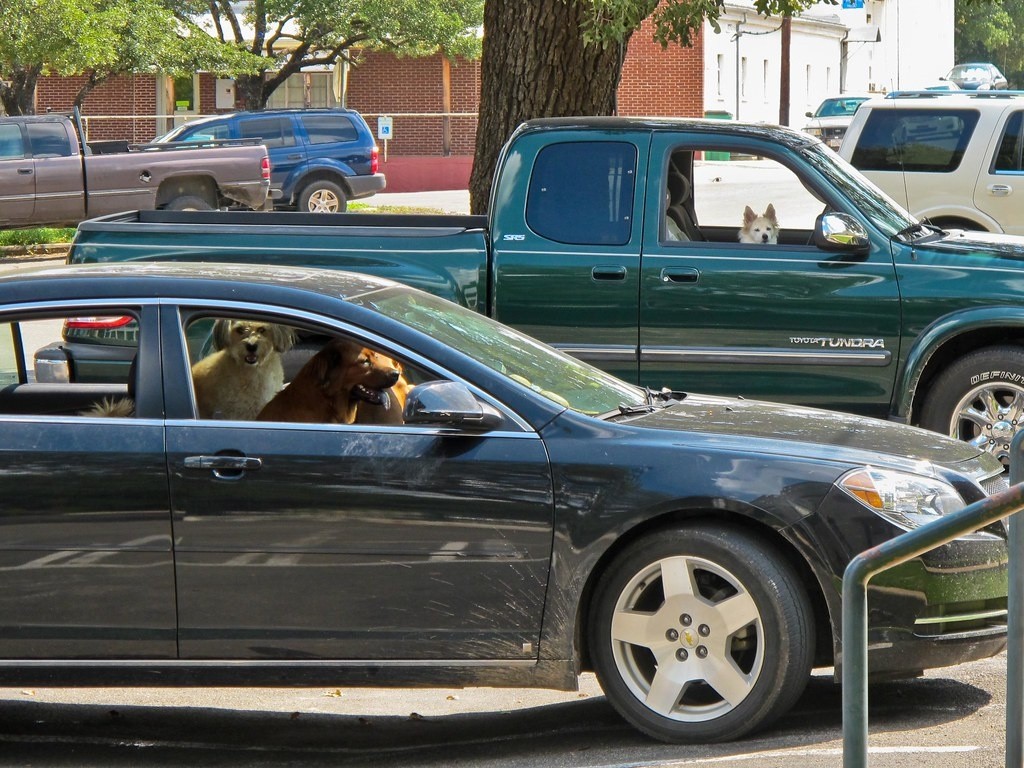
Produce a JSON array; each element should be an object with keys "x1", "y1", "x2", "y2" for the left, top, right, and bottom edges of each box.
[{"x1": 662, "y1": 171, "x2": 712, "y2": 244}]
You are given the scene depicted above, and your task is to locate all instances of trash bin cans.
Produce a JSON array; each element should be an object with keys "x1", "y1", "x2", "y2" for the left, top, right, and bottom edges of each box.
[{"x1": 703, "y1": 109, "x2": 733, "y2": 162}]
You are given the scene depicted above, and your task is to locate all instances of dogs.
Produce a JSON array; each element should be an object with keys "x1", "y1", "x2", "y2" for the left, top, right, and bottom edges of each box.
[
  {"x1": 76, "y1": 319, "x2": 416, "y2": 427},
  {"x1": 737, "y1": 203, "x2": 780, "y2": 245}
]
